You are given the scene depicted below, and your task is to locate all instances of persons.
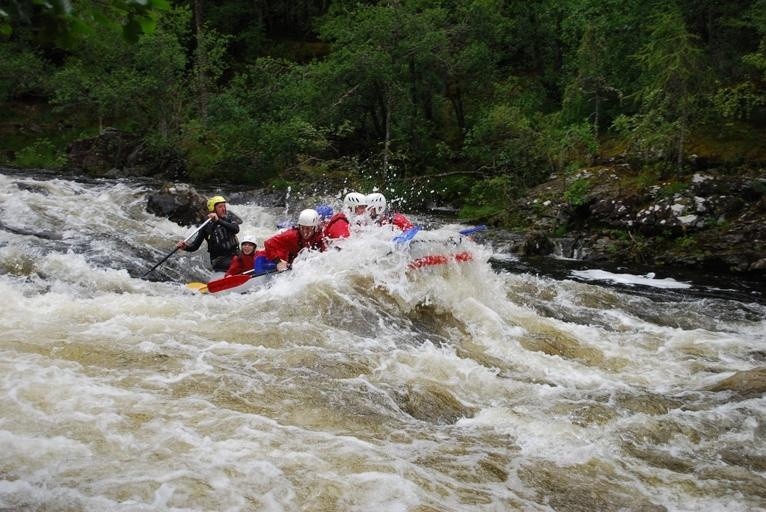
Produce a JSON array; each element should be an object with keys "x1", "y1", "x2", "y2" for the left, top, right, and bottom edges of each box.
[
  {"x1": 223, "y1": 192, "x2": 413, "y2": 278},
  {"x1": 176, "y1": 194, "x2": 243, "y2": 272}
]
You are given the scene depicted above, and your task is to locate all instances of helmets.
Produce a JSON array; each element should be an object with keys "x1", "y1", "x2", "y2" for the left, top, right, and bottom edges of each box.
[
  {"x1": 241, "y1": 234, "x2": 258, "y2": 248},
  {"x1": 363, "y1": 191, "x2": 387, "y2": 215},
  {"x1": 343, "y1": 191, "x2": 368, "y2": 210},
  {"x1": 298, "y1": 207, "x2": 319, "y2": 227},
  {"x1": 315, "y1": 203, "x2": 333, "y2": 220},
  {"x1": 206, "y1": 195, "x2": 225, "y2": 213}
]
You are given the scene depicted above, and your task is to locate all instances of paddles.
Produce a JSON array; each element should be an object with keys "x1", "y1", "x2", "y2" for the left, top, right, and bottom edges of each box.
[
  {"x1": 391, "y1": 224, "x2": 421, "y2": 243},
  {"x1": 457, "y1": 225, "x2": 487, "y2": 236},
  {"x1": 254, "y1": 255, "x2": 292, "y2": 276},
  {"x1": 207, "y1": 269, "x2": 280, "y2": 293},
  {"x1": 182, "y1": 268, "x2": 254, "y2": 293}
]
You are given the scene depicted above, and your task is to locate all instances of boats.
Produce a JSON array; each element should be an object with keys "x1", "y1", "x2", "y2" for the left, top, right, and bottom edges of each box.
[{"x1": 187, "y1": 239, "x2": 474, "y2": 297}]
